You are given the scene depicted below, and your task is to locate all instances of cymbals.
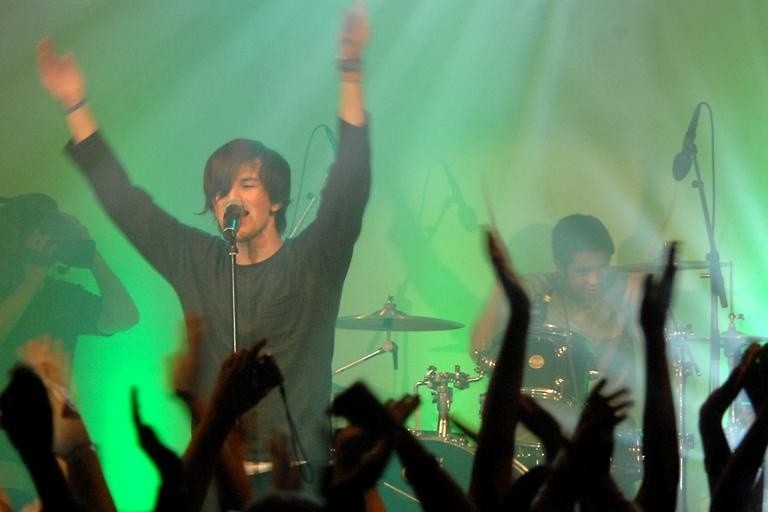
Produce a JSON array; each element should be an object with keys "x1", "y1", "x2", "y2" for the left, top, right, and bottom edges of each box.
[
  {"x1": 607, "y1": 261, "x2": 706, "y2": 272},
  {"x1": 693, "y1": 336, "x2": 766, "y2": 346},
  {"x1": 337, "y1": 310, "x2": 467, "y2": 329}
]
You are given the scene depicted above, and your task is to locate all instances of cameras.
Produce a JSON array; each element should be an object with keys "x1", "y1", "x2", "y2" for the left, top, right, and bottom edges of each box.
[{"x1": 39, "y1": 213, "x2": 95, "y2": 269}]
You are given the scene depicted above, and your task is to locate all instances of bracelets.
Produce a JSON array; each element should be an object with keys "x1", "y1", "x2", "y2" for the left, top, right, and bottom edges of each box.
[
  {"x1": 340, "y1": 57, "x2": 365, "y2": 73},
  {"x1": 64, "y1": 95, "x2": 89, "y2": 113}
]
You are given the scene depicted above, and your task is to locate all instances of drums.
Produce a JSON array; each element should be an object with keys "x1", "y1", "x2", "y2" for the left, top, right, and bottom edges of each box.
[
  {"x1": 490, "y1": 326, "x2": 604, "y2": 450},
  {"x1": 609, "y1": 435, "x2": 645, "y2": 480},
  {"x1": 376, "y1": 432, "x2": 547, "y2": 512}
]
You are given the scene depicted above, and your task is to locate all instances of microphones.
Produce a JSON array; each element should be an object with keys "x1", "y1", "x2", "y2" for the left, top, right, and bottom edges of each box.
[
  {"x1": 223, "y1": 200, "x2": 243, "y2": 241},
  {"x1": 672, "y1": 103, "x2": 700, "y2": 179},
  {"x1": 325, "y1": 126, "x2": 337, "y2": 152}
]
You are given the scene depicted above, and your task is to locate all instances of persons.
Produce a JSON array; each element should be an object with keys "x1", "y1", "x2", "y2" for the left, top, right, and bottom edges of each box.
[
  {"x1": 34, "y1": 1, "x2": 374, "y2": 485},
  {"x1": 0, "y1": 190, "x2": 141, "y2": 508},
  {"x1": 471, "y1": 221, "x2": 683, "y2": 509},
  {"x1": 469, "y1": 213, "x2": 646, "y2": 425},
  {"x1": 1, "y1": 329, "x2": 767, "y2": 510}
]
